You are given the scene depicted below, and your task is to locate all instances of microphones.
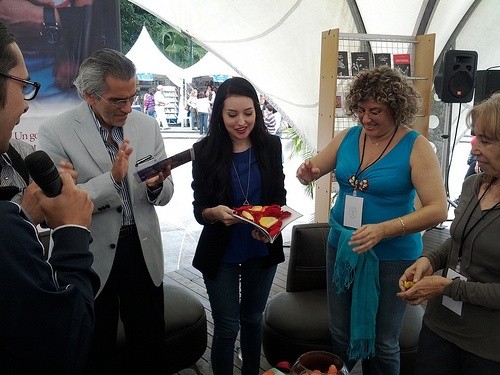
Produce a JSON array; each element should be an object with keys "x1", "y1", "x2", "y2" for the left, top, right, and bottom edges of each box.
[{"x1": 24, "y1": 150, "x2": 94, "y2": 245}]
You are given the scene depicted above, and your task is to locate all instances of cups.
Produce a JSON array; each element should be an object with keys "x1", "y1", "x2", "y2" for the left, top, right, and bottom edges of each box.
[{"x1": 290, "y1": 351, "x2": 350, "y2": 375}]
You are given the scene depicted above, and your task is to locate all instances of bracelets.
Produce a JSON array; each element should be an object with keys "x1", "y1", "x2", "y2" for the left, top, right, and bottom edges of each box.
[{"x1": 398, "y1": 216, "x2": 406, "y2": 236}]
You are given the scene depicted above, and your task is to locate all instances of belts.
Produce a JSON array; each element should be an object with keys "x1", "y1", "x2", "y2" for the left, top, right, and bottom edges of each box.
[{"x1": 119, "y1": 227, "x2": 137, "y2": 238}]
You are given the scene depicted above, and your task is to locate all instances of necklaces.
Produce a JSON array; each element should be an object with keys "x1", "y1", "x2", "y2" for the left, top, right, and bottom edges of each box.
[
  {"x1": 367, "y1": 135, "x2": 380, "y2": 148},
  {"x1": 231, "y1": 140, "x2": 252, "y2": 205}
]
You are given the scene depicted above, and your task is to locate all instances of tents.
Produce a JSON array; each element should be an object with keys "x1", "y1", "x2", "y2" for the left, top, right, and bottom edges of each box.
[{"x1": 123, "y1": 25, "x2": 243, "y2": 129}]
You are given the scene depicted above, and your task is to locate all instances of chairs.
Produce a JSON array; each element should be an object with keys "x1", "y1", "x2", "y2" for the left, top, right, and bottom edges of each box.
[{"x1": 118, "y1": 285, "x2": 207, "y2": 375}]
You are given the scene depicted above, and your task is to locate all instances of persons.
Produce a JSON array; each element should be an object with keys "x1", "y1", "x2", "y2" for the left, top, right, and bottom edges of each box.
[
  {"x1": 191, "y1": 77, "x2": 289, "y2": 375},
  {"x1": 397, "y1": 90, "x2": 500, "y2": 375},
  {"x1": 0, "y1": 47, "x2": 174, "y2": 375},
  {"x1": 187, "y1": 84, "x2": 283, "y2": 136},
  {"x1": 296, "y1": 65, "x2": 448, "y2": 375}
]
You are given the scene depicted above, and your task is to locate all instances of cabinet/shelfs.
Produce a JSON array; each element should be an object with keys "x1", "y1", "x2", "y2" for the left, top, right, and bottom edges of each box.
[
  {"x1": 315, "y1": 28, "x2": 436, "y2": 223},
  {"x1": 162, "y1": 86, "x2": 179, "y2": 124}
]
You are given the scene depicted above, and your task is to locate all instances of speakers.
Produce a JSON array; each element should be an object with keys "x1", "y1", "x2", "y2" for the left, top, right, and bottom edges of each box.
[
  {"x1": 471, "y1": 70, "x2": 500, "y2": 136},
  {"x1": 434, "y1": 49, "x2": 478, "y2": 103}
]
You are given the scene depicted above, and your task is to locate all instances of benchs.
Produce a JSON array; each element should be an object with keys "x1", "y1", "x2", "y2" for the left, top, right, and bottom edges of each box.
[{"x1": 262, "y1": 223, "x2": 452, "y2": 375}]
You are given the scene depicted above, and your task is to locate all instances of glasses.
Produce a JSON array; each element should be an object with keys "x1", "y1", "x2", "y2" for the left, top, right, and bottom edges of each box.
[
  {"x1": 352, "y1": 107, "x2": 390, "y2": 120},
  {"x1": 0, "y1": 72, "x2": 40, "y2": 100},
  {"x1": 94, "y1": 91, "x2": 140, "y2": 108}
]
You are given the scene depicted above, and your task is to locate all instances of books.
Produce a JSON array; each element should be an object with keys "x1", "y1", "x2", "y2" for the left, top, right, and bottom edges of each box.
[{"x1": 232, "y1": 205, "x2": 303, "y2": 243}]
